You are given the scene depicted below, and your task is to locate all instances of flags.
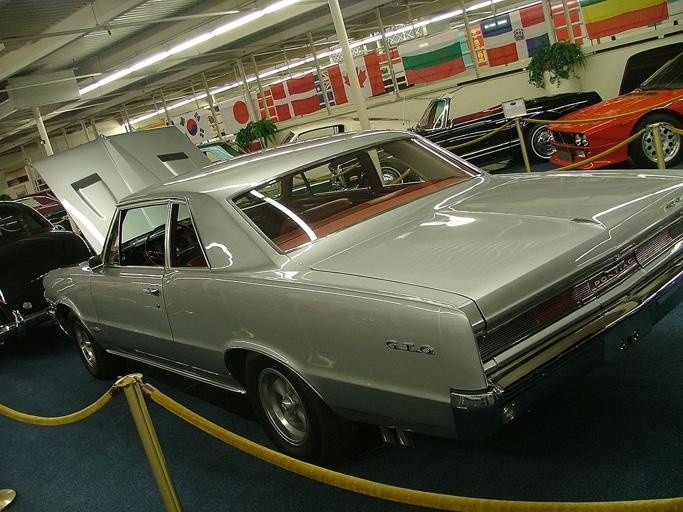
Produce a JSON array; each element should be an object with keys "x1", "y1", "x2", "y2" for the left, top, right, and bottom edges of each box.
[
  {"x1": 578, "y1": 0, "x2": 670, "y2": 41},
  {"x1": 218, "y1": 90, "x2": 261, "y2": 135},
  {"x1": 396, "y1": 30, "x2": 465, "y2": 86},
  {"x1": 479, "y1": 0, "x2": 559, "y2": 67},
  {"x1": 170, "y1": 108, "x2": 213, "y2": 145},
  {"x1": 269, "y1": 72, "x2": 322, "y2": 122},
  {"x1": 327, "y1": 51, "x2": 385, "y2": 105}
]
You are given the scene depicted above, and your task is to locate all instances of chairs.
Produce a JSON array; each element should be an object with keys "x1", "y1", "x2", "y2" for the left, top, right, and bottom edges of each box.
[{"x1": 253, "y1": 196, "x2": 353, "y2": 234}]
